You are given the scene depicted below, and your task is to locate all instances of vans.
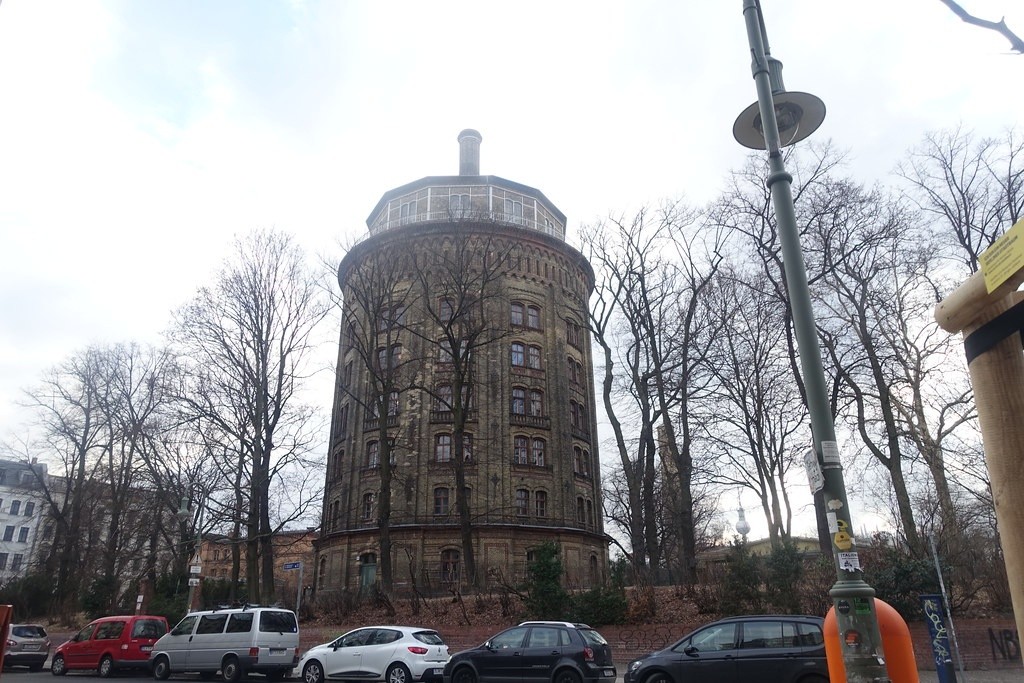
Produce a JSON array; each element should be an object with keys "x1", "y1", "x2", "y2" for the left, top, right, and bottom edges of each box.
[
  {"x1": 51, "y1": 615, "x2": 169, "y2": 678},
  {"x1": 149, "y1": 604, "x2": 300, "y2": 683}
]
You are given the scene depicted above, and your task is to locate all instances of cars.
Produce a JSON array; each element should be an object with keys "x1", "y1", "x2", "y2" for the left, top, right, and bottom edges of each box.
[
  {"x1": 2, "y1": 623, "x2": 51, "y2": 672},
  {"x1": 292, "y1": 625, "x2": 450, "y2": 683}
]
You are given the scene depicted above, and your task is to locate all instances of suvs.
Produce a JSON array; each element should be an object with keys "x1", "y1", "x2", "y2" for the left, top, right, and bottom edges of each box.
[
  {"x1": 623, "y1": 614, "x2": 829, "y2": 682},
  {"x1": 441, "y1": 620, "x2": 617, "y2": 683}
]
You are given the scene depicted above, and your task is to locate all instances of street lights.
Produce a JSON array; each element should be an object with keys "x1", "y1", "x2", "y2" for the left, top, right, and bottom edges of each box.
[{"x1": 732, "y1": 0, "x2": 891, "y2": 682}]
